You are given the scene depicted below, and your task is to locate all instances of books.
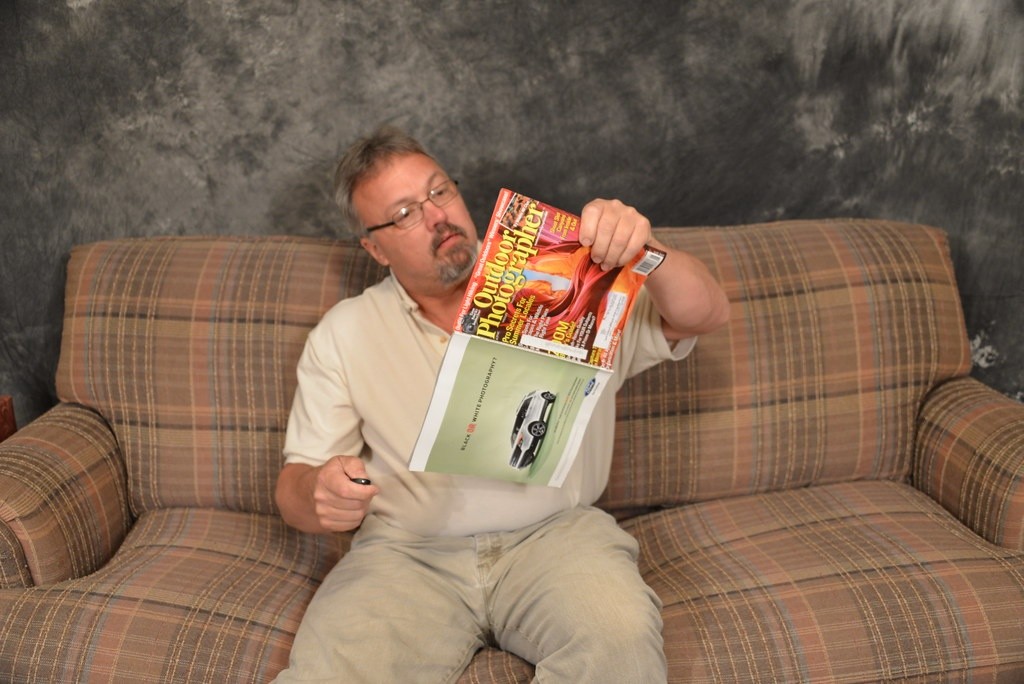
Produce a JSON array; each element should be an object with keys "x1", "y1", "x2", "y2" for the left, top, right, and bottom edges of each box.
[{"x1": 408, "y1": 188, "x2": 667, "y2": 488}]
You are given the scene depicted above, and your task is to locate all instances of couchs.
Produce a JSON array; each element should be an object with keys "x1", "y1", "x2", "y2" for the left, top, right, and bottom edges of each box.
[{"x1": 0, "y1": 218, "x2": 1024, "y2": 684}]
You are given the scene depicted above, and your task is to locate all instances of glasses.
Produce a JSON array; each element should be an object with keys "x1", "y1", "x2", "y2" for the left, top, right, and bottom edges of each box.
[{"x1": 366, "y1": 179, "x2": 459, "y2": 234}]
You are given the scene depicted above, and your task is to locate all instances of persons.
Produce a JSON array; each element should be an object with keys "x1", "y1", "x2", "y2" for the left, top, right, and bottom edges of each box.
[{"x1": 269, "y1": 130, "x2": 730, "y2": 684}]
